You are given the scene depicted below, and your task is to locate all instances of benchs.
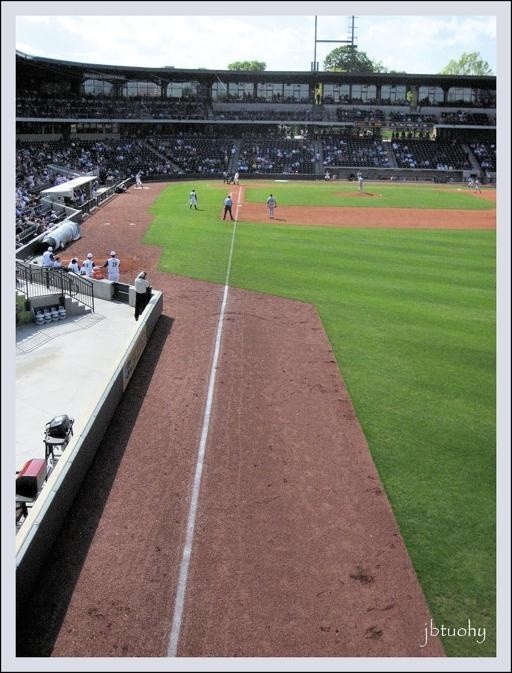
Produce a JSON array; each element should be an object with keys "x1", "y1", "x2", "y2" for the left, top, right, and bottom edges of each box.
[{"x1": 15, "y1": 199, "x2": 67, "y2": 244}]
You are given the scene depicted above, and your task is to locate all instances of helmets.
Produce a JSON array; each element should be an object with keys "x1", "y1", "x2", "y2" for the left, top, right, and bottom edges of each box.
[
  {"x1": 110, "y1": 251, "x2": 116, "y2": 255},
  {"x1": 87, "y1": 253, "x2": 92, "y2": 257},
  {"x1": 139, "y1": 272, "x2": 146, "y2": 277},
  {"x1": 48, "y1": 247, "x2": 52, "y2": 251}
]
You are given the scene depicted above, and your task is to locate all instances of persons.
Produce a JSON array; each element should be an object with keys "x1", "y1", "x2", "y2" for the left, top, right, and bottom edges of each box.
[{"x1": 16, "y1": 91, "x2": 495, "y2": 321}]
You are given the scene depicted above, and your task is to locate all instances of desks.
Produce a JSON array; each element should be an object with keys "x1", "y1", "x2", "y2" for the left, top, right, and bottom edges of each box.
[
  {"x1": 16, "y1": 465, "x2": 51, "y2": 523},
  {"x1": 43, "y1": 418, "x2": 75, "y2": 463}
]
